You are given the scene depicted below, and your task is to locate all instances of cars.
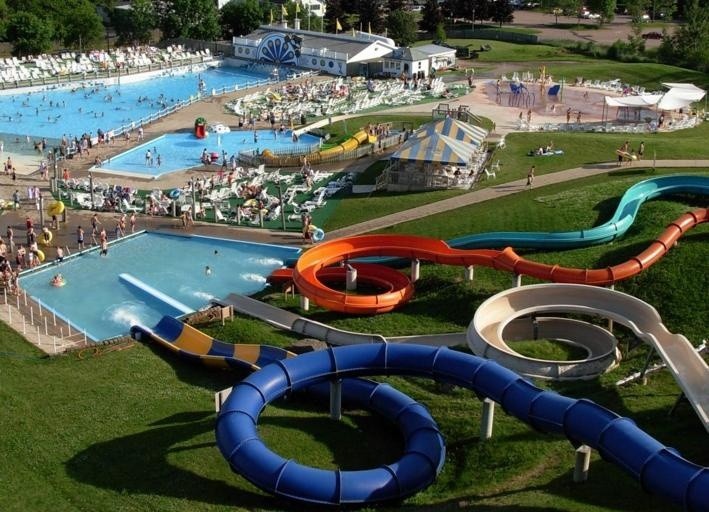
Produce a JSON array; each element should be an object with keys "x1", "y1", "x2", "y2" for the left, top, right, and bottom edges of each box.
[
  {"x1": 507, "y1": 0, "x2": 665, "y2": 22},
  {"x1": 408, "y1": 0, "x2": 445, "y2": 12},
  {"x1": 642, "y1": 31, "x2": 668, "y2": 40}
]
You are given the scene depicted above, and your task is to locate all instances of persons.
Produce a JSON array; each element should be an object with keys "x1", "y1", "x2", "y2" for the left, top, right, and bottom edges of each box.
[
  {"x1": 237, "y1": 79, "x2": 335, "y2": 140},
  {"x1": 400, "y1": 68, "x2": 437, "y2": 91},
  {"x1": 496, "y1": 64, "x2": 698, "y2": 186},
  {"x1": 366, "y1": 122, "x2": 392, "y2": 139},
  {"x1": 0, "y1": 183, "x2": 137, "y2": 296},
  {"x1": 124, "y1": 67, "x2": 206, "y2": 144},
  {"x1": 140, "y1": 146, "x2": 317, "y2": 246},
  {"x1": 1, "y1": 65, "x2": 131, "y2": 181}
]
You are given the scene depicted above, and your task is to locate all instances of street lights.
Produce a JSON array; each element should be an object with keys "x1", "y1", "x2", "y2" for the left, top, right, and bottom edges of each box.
[
  {"x1": 37, "y1": 192, "x2": 45, "y2": 230},
  {"x1": 88, "y1": 170, "x2": 96, "y2": 211},
  {"x1": 187, "y1": 177, "x2": 198, "y2": 221},
  {"x1": 649, "y1": 127, "x2": 659, "y2": 168},
  {"x1": 52, "y1": 146, "x2": 58, "y2": 191},
  {"x1": 472, "y1": 6, "x2": 475, "y2": 30},
  {"x1": 274, "y1": 184, "x2": 286, "y2": 232}
]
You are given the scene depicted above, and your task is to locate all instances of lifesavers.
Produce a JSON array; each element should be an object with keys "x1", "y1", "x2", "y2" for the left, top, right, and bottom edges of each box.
[
  {"x1": 48, "y1": 276, "x2": 68, "y2": 288},
  {"x1": 169, "y1": 189, "x2": 180, "y2": 199},
  {"x1": 40, "y1": 231, "x2": 52, "y2": 245},
  {"x1": 36, "y1": 250, "x2": 44, "y2": 262},
  {"x1": 615, "y1": 149, "x2": 638, "y2": 160},
  {"x1": 302, "y1": 225, "x2": 316, "y2": 239},
  {"x1": 312, "y1": 229, "x2": 325, "y2": 244}
]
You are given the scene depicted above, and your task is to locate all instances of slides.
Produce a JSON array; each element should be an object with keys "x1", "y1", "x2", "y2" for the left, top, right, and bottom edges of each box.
[
  {"x1": 47, "y1": 201, "x2": 65, "y2": 217},
  {"x1": 283, "y1": 175, "x2": 709, "y2": 268},
  {"x1": 131, "y1": 315, "x2": 297, "y2": 374},
  {"x1": 208, "y1": 282, "x2": 708, "y2": 432},
  {"x1": 197, "y1": 124, "x2": 207, "y2": 138},
  {"x1": 266, "y1": 233, "x2": 512, "y2": 314}
]
